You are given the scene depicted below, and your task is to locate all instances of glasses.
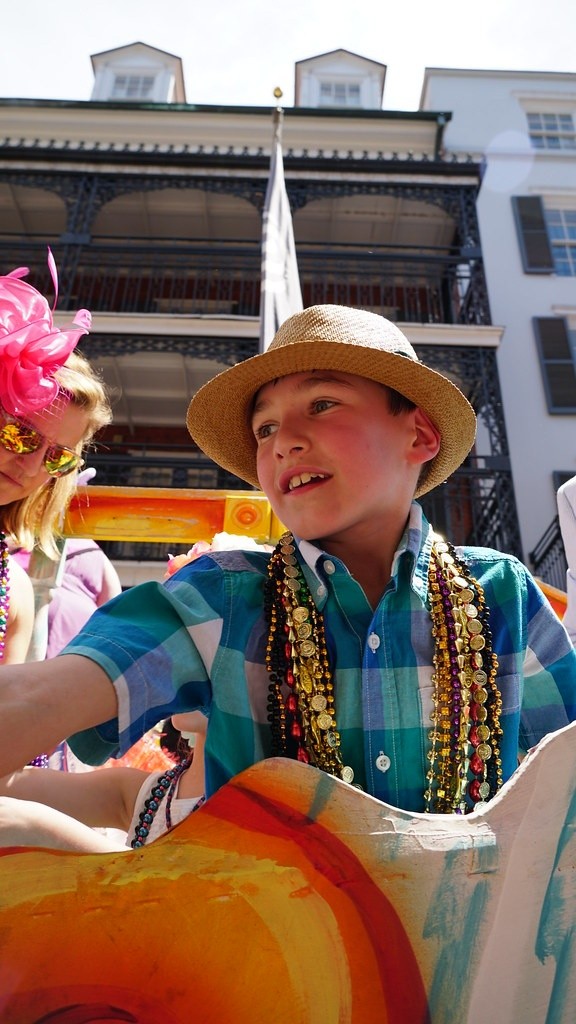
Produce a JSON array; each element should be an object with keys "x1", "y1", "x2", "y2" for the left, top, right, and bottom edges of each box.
[{"x1": 0, "y1": 404, "x2": 85, "y2": 478}]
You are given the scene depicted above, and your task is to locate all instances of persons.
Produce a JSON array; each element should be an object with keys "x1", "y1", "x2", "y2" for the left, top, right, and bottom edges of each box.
[
  {"x1": 0, "y1": 300, "x2": 576, "y2": 866},
  {"x1": 0, "y1": 707, "x2": 209, "y2": 853},
  {"x1": 0, "y1": 351, "x2": 121, "y2": 774}
]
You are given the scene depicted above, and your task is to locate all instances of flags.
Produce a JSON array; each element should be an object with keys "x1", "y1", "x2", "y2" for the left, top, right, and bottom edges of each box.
[{"x1": 259, "y1": 143, "x2": 305, "y2": 351}]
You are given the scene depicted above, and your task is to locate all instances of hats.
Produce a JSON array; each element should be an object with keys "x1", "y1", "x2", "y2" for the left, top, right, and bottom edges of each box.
[{"x1": 186, "y1": 304, "x2": 477, "y2": 500}]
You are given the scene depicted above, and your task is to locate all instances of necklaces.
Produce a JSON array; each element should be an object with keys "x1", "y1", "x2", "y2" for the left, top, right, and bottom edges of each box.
[
  {"x1": 131, "y1": 758, "x2": 205, "y2": 850},
  {"x1": 266, "y1": 529, "x2": 503, "y2": 813},
  {"x1": 0, "y1": 532, "x2": 9, "y2": 660}
]
